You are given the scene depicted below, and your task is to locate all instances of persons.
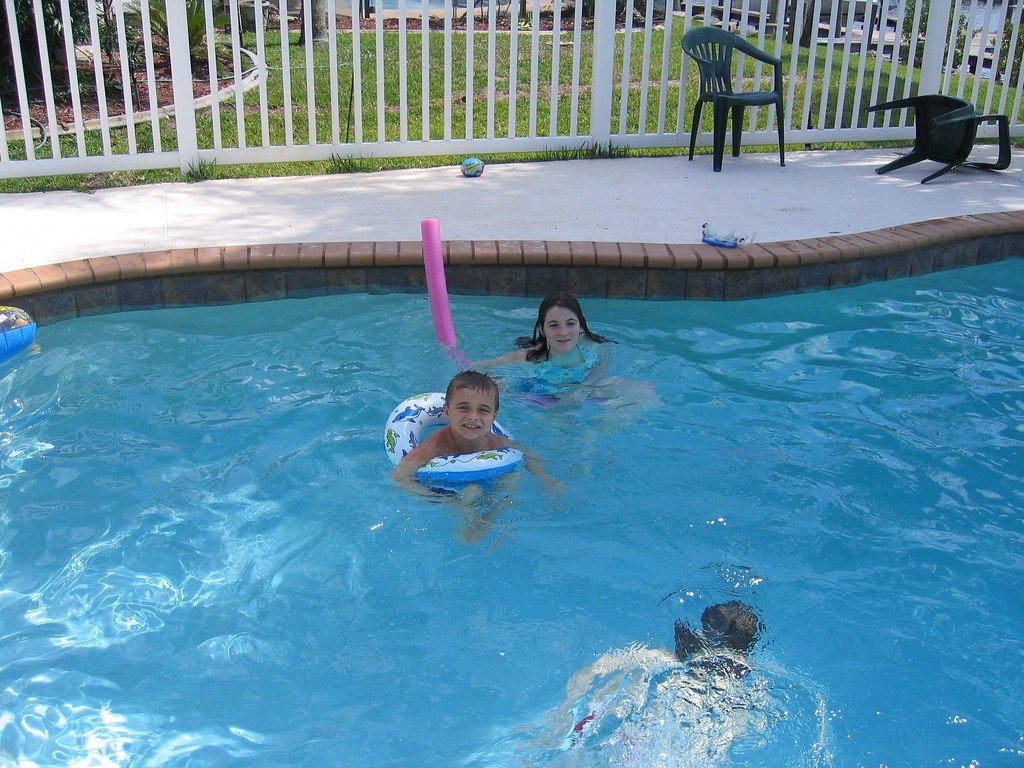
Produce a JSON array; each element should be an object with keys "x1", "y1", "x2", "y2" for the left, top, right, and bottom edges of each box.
[
  {"x1": 497, "y1": 291, "x2": 619, "y2": 360},
  {"x1": 402, "y1": 370, "x2": 507, "y2": 463}
]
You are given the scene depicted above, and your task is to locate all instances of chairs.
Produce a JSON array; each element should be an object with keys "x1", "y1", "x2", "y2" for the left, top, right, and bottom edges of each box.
[
  {"x1": 681, "y1": 26, "x2": 786, "y2": 172},
  {"x1": 865, "y1": 92, "x2": 1012, "y2": 184}
]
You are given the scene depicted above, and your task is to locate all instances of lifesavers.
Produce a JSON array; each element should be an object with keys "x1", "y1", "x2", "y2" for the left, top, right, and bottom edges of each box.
[
  {"x1": 418, "y1": 216, "x2": 607, "y2": 408},
  {"x1": 381, "y1": 391, "x2": 526, "y2": 485},
  {"x1": 0, "y1": 303, "x2": 39, "y2": 367}
]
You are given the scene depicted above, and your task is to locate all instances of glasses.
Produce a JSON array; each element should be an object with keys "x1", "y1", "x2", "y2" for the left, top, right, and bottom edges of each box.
[{"x1": 702, "y1": 222, "x2": 756, "y2": 249}]
